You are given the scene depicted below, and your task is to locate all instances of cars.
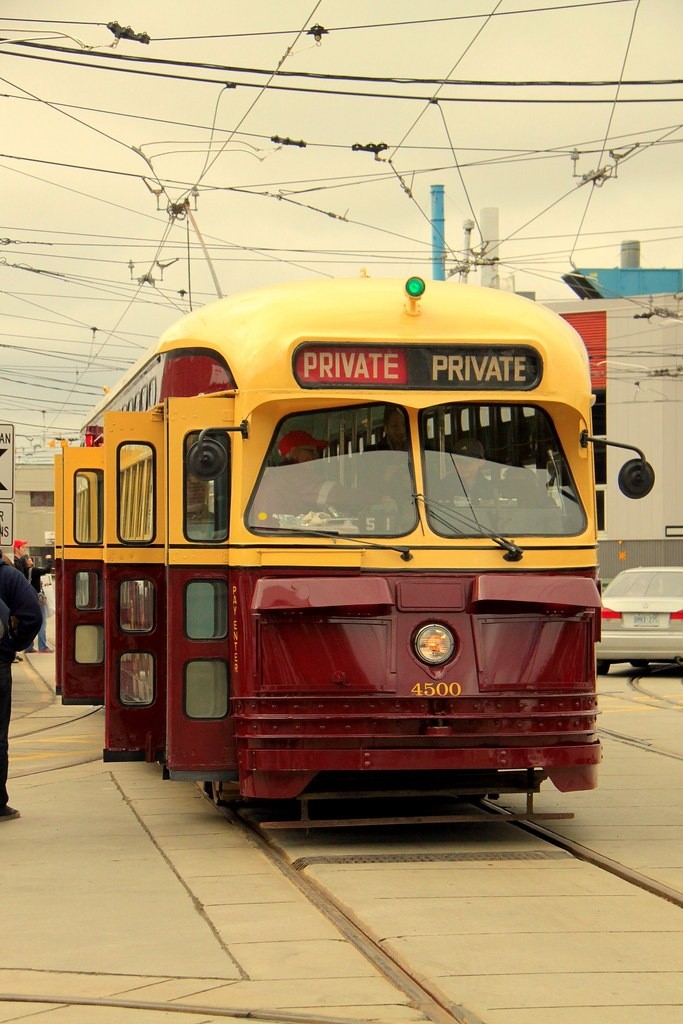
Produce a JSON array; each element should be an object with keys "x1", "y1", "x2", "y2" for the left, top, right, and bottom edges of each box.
[{"x1": 598, "y1": 564, "x2": 683, "y2": 678}]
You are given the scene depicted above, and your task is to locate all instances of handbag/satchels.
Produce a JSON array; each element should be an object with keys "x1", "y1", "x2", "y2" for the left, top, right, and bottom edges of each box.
[{"x1": 37, "y1": 591, "x2": 46, "y2": 606}]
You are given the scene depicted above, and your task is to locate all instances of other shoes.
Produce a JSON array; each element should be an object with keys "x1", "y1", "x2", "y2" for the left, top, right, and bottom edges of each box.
[
  {"x1": 26, "y1": 647, "x2": 37, "y2": 653},
  {"x1": 14, "y1": 655, "x2": 23, "y2": 663},
  {"x1": 0, "y1": 804, "x2": 20, "y2": 821},
  {"x1": 39, "y1": 648, "x2": 55, "y2": 653}
]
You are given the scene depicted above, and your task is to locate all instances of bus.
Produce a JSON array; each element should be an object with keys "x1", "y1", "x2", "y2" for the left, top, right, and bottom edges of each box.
[{"x1": 41, "y1": 277, "x2": 662, "y2": 835}]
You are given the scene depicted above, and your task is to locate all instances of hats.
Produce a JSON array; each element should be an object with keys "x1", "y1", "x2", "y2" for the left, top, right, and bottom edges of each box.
[
  {"x1": 277, "y1": 431, "x2": 329, "y2": 455},
  {"x1": 14, "y1": 539, "x2": 28, "y2": 547},
  {"x1": 448, "y1": 439, "x2": 484, "y2": 460}
]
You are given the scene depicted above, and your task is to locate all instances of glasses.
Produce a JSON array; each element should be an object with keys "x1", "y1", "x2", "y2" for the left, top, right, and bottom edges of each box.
[{"x1": 298, "y1": 447, "x2": 319, "y2": 454}]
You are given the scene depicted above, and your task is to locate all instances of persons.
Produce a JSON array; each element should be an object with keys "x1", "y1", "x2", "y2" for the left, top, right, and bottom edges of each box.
[
  {"x1": 11, "y1": 539, "x2": 30, "y2": 663},
  {"x1": 20, "y1": 553, "x2": 56, "y2": 654},
  {"x1": 245, "y1": 402, "x2": 565, "y2": 537},
  {"x1": 0, "y1": 550, "x2": 43, "y2": 824}
]
